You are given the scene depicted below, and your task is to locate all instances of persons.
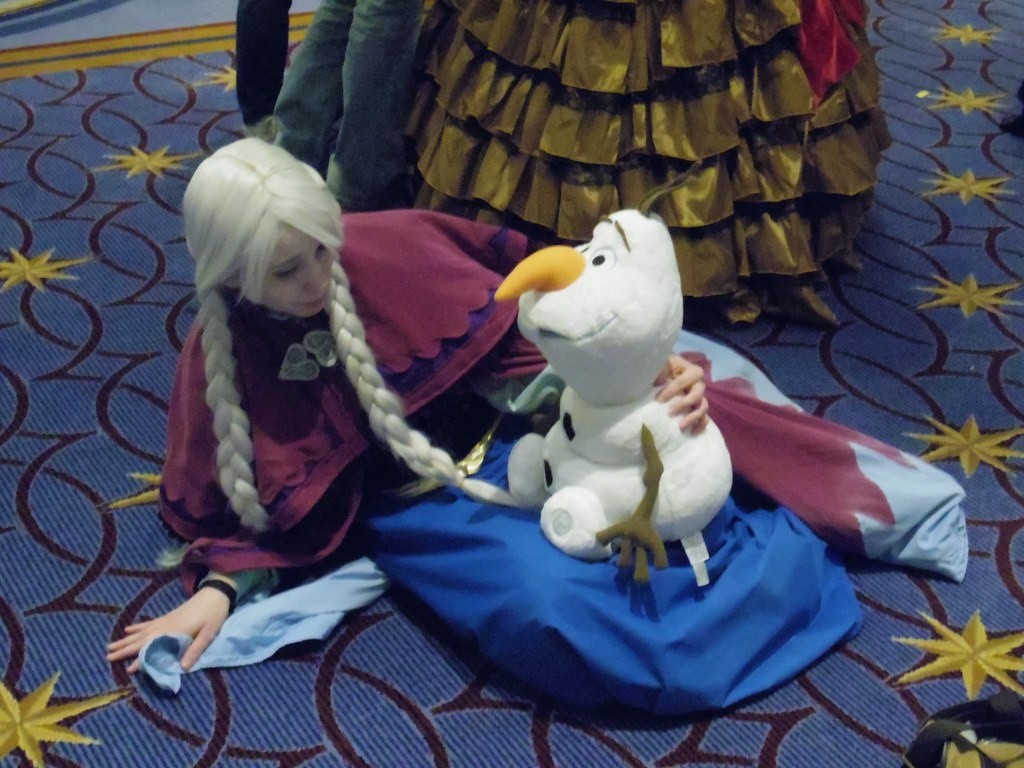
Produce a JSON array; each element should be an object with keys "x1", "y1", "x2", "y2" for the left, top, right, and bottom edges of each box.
[
  {"x1": 272, "y1": 2, "x2": 420, "y2": 208},
  {"x1": 236, "y1": 1, "x2": 290, "y2": 143},
  {"x1": 409, "y1": 0, "x2": 897, "y2": 326},
  {"x1": 106, "y1": 137, "x2": 969, "y2": 713}
]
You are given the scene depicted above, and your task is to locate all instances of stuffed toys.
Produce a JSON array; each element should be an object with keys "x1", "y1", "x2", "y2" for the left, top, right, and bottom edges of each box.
[{"x1": 493, "y1": 160, "x2": 733, "y2": 583}]
